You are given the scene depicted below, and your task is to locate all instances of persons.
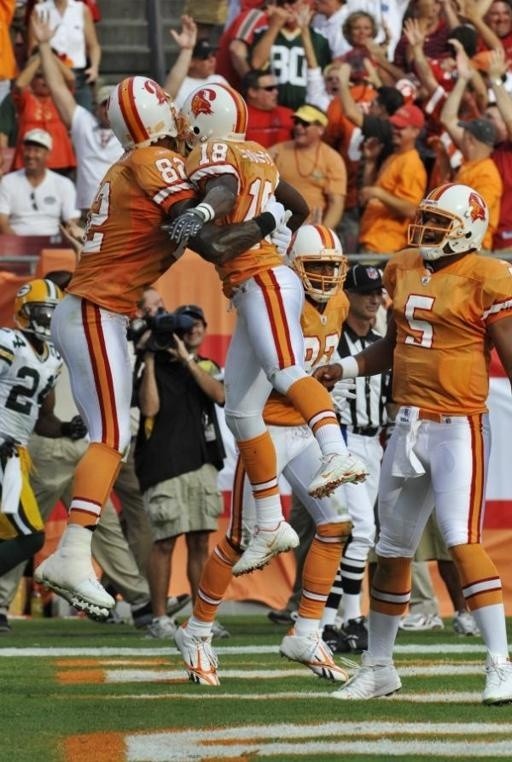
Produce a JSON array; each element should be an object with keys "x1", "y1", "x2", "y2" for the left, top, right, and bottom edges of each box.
[
  {"x1": 175, "y1": 225, "x2": 352, "y2": 690},
  {"x1": 1, "y1": 0, "x2": 512, "y2": 652},
  {"x1": 310, "y1": 183, "x2": 512, "y2": 705}
]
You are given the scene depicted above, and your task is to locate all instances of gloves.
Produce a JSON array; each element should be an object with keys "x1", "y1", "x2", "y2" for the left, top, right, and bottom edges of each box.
[
  {"x1": 161, "y1": 202, "x2": 215, "y2": 243},
  {"x1": 262, "y1": 193, "x2": 294, "y2": 229}
]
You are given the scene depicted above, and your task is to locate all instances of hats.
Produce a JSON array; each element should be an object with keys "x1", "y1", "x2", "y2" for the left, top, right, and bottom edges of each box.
[
  {"x1": 95, "y1": 85, "x2": 117, "y2": 106},
  {"x1": 291, "y1": 105, "x2": 329, "y2": 127},
  {"x1": 457, "y1": 118, "x2": 497, "y2": 147},
  {"x1": 22, "y1": 127, "x2": 54, "y2": 151},
  {"x1": 194, "y1": 37, "x2": 219, "y2": 56},
  {"x1": 388, "y1": 104, "x2": 423, "y2": 128},
  {"x1": 343, "y1": 264, "x2": 383, "y2": 295},
  {"x1": 174, "y1": 305, "x2": 207, "y2": 327}
]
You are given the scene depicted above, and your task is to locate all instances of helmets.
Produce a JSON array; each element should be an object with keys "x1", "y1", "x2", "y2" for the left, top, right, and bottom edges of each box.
[
  {"x1": 12, "y1": 277, "x2": 66, "y2": 341},
  {"x1": 180, "y1": 83, "x2": 249, "y2": 149},
  {"x1": 407, "y1": 182, "x2": 490, "y2": 262},
  {"x1": 106, "y1": 75, "x2": 178, "y2": 155},
  {"x1": 287, "y1": 224, "x2": 348, "y2": 304}
]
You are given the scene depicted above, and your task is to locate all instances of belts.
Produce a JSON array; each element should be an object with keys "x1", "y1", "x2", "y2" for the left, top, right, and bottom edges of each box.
[{"x1": 340, "y1": 424, "x2": 378, "y2": 436}]
